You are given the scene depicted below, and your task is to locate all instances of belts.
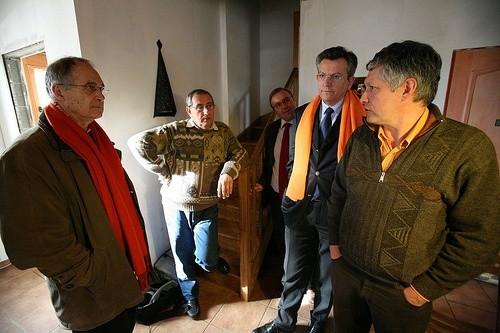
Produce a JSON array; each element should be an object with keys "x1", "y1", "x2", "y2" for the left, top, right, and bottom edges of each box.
[{"x1": 313, "y1": 196, "x2": 322, "y2": 201}]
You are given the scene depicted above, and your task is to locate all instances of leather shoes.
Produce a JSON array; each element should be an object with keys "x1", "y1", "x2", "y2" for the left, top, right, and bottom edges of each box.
[
  {"x1": 214, "y1": 257, "x2": 230, "y2": 274},
  {"x1": 252, "y1": 320, "x2": 287, "y2": 333},
  {"x1": 306, "y1": 325, "x2": 324, "y2": 333},
  {"x1": 186, "y1": 297, "x2": 200, "y2": 318}
]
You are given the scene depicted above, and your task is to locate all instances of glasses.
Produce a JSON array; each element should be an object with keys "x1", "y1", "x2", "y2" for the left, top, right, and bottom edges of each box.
[
  {"x1": 317, "y1": 72, "x2": 351, "y2": 82},
  {"x1": 273, "y1": 96, "x2": 293, "y2": 109},
  {"x1": 189, "y1": 104, "x2": 216, "y2": 112},
  {"x1": 55, "y1": 83, "x2": 106, "y2": 98}
]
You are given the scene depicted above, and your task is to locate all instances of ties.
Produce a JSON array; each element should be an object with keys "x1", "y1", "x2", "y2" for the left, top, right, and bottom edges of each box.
[
  {"x1": 321, "y1": 108, "x2": 334, "y2": 139},
  {"x1": 279, "y1": 122, "x2": 293, "y2": 198}
]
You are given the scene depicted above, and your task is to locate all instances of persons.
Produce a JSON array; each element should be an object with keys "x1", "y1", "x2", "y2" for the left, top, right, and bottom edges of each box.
[
  {"x1": 252, "y1": 46, "x2": 366, "y2": 333},
  {"x1": 0, "y1": 57, "x2": 162, "y2": 333},
  {"x1": 129, "y1": 89, "x2": 248, "y2": 320},
  {"x1": 327, "y1": 42, "x2": 500, "y2": 333},
  {"x1": 255, "y1": 88, "x2": 296, "y2": 297}
]
